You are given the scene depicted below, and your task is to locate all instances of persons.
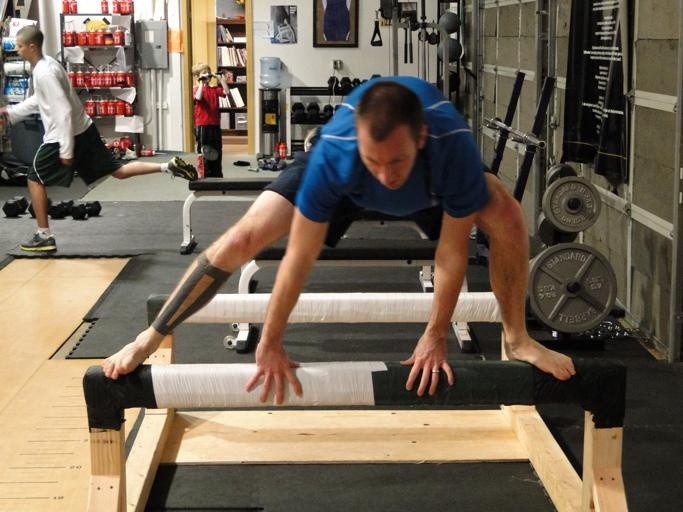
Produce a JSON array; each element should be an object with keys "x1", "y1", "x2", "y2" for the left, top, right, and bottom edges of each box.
[
  {"x1": 102, "y1": 78, "x2": 576, "y2": 405},
  {"x1": 191, "y1": 64, "x2": 229, "y2": 177},
  {"x1": 0, "y1": 26, "x2": 199, "y2": 251}
]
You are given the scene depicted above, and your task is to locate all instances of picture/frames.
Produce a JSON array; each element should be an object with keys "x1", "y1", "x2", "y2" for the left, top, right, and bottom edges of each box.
[{"x1": 313, "y1": 0, "x2": 360, "y2": 48}]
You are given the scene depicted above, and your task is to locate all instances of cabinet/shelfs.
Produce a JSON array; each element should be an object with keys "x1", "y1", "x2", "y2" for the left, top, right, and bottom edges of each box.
[
  {"x1": 286, "y1": 87, "x2": 354, "y2": 160},
  {"x1": 216, "y1": 17, "x2": 248, "y2": 136},
  {"x1": 59, "y1": 13, "x2": 140, "y2": 160}
]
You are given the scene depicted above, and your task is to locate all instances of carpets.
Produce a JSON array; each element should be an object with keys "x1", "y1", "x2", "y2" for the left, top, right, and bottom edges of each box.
[
  {"x1": 0, "y1": 174, "x2": 111, "y2": 270},
  {"x1": 7, "y1": 200, "x2": 683, "y2": 512}
]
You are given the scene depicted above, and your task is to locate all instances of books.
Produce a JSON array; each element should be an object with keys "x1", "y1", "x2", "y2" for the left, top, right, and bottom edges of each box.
[
  {"x1": 216, "y1": 46, "x2": 246, "y2": 67},
  {"x1": 218, "y1": 85, "x2": 247, "y2": 109},
  {"x1": 216, "y1": 23, "x2": 246, "y2": 44}
]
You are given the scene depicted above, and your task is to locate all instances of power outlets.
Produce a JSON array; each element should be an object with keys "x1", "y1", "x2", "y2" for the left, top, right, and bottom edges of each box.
[{"x1": 333, "y1": 60, "x2": 343, "y2": 70}]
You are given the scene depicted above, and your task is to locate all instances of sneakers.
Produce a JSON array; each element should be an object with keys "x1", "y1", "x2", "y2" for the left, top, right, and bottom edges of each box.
[
  {"x1": 122, "y1": 147, "x2": 137, "y2": 160},
  {"x1": 20, "y1": 233, "x2": 57, "y2": 251},
  {"x1": 166, "y1": 156, "x2": 198, "y2": 181}
]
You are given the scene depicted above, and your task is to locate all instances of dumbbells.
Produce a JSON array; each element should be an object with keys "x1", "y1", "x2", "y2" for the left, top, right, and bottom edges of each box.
[
  {"x1": 292, "y1": 102, "x2": 340, "y2": 122},
  {"x1": 2, "y1": 196, "x2": 28, "y2": 218},
  {"x1": 328, "y1": 75, "x2": 381, "y2": 91},
  {"x1": 28, "y1": 197, "x2": 101, "y2": 220}
]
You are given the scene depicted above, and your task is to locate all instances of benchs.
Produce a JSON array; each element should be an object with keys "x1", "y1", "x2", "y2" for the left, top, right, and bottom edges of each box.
[
  {"x1": 180, "y1": 176, "x2": 434, "y2": 292},
  {"x1": 222, "y1": 239, "x2": 478, "y2": 354}
]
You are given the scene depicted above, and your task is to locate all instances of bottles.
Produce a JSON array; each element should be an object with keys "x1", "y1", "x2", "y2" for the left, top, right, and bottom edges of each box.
[
  {"x1": 68, "y1": 70, "x2": 135, "y2": 87},
  {"x1": 279, "y1": 141, "x2": 286, "y2": 159},
  {"x1": 62, "y1": 0, "x2": 77, "y2": 14},
  {"x1": 4, "y1": 77, "x2": 28, "y2": 95},
  {"x1": 83, "y1": 97, "x2": 134, "y2": 116},
  {"x1": 4, "y1": 39, "x2": 10, "y2": 49},
  {"x1": 62, "y1": 26, "x2": 131, "y2": 46},
  {"x1": 139, "y1": 149, "x2": 156, "y2": 157},
  {"x1": 101, "y1": 0, "x2": 133, "y2": 15}
]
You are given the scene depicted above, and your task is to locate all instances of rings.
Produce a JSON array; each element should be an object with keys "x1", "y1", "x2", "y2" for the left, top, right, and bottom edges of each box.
[{"x1": 432, "y1": 369, "x2": 440, "y2": 373}]
